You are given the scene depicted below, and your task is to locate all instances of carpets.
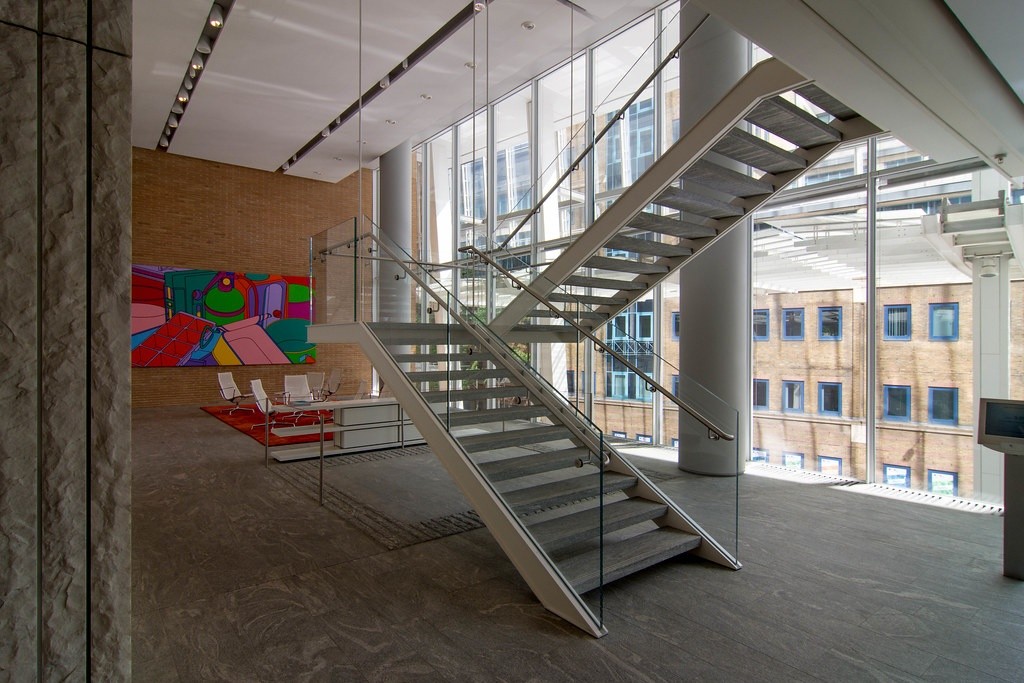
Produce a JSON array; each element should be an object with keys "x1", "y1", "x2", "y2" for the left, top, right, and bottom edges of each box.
[
  {"x1": 271, "y1": 437, "x2": 623, "y2": 551},
  {"x1": 199, "y1": 400, "x2": 336, "y2": 446}
]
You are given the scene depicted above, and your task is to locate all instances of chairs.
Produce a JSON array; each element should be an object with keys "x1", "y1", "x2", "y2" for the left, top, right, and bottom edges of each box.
[{"x1": 217, "y1": 366, "x2": 345, "y2": 430}]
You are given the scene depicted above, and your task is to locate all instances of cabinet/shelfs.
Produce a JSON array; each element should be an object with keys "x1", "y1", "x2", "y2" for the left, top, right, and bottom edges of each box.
[{"x1": 264, "y1": 396, "x2": 446, "y2": 469}]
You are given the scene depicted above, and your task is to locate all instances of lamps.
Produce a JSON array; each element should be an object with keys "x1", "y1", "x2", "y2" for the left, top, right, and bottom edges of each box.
[
  {"x1": 160, "y1": 37, "x2": 211, "y2": 148},
  {"x1": 281, "y1": 1, "x2": 485, "y2": 171},
  {"x1": 210, "y1": 3, "x2": 223, "y2": 28}
]
